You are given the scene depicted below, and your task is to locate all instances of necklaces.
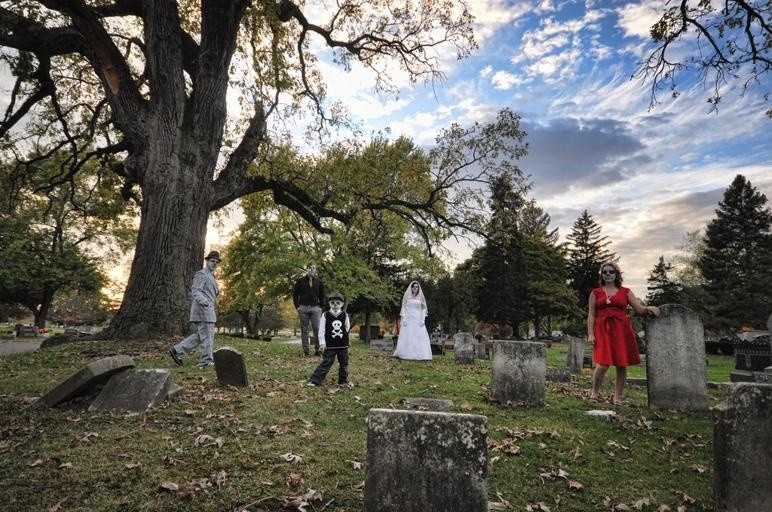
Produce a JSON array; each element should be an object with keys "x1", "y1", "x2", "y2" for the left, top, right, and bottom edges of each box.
[{"x1": 605, "y1": 285, "x2": 617, "y2": 304}]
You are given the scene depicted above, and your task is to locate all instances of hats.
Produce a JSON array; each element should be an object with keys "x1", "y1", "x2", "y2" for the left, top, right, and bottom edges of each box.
[
  {"x1": 205, "y1": 251, "x2": 221, "y2": 262},
  {"x1": 328, "y1": 292, "x2": 344, "y2": 302}
]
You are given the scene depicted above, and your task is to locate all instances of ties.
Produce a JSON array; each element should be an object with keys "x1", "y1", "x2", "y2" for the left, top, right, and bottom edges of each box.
[{"x1": 309, "y1": 276, "x2": 312, "y2": 288}]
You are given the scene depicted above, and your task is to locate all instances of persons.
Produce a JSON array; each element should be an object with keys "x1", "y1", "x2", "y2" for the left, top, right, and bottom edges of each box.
[
  {"x1": 588, "y1": 261, "x2": 660, "y2": 406},
  {"x1": 475, "y1": 329, "x2": 482, "y2": 342},
  {"x1": 293, "y1": 263, "x2": 324, "y2": 356},
  {"x1": 170, "y1": 250, "x2": 223, "y2": 366},
  {"x1": 392, "y1": 281, "x2": 432, "y2": 361},
  {"x1": 306, "y1": 291, "x2": 350, "y2": 388}
]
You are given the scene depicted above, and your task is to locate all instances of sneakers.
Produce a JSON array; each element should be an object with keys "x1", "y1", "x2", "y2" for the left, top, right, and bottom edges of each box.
[
  {"x1": 169, "y1": 347, "x2": 183, "y2": 366},
  {"x1": 302, "y1": 351, "x2": 323, "y2": 357},
  {"x1": 340, "y1": 383, "x2": 354, "y2": 387},
  {"x1": 197, "y1": 362, "x2": 215, "y2": 368},
  {"x1": 306, "y1": 381, "x2": 316, "y2": 387}
]
